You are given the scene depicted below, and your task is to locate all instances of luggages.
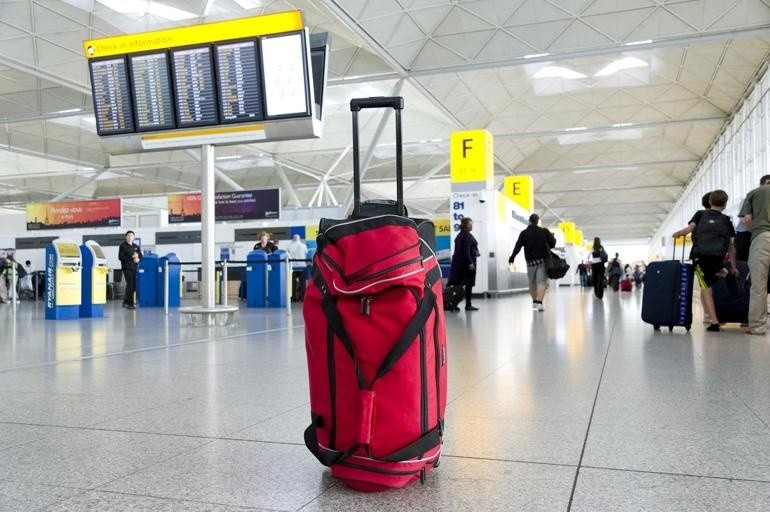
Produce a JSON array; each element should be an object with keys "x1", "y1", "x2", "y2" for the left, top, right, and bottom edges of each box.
[
  {"x1": 713, "y1": 261, "x2": 749, "y2": 324},
  {"x1": 303, "y1": 97, "x2": 446, "y2": 491},
  {"x1": 641, "y1": 235, "x2": 692, "y2": 331},
  {"x1": 443, "y1": 268, "x2": 476, "y2": 310},
  {"x1": 621, "y1": 274, "x2": 632, "y2": 291}
]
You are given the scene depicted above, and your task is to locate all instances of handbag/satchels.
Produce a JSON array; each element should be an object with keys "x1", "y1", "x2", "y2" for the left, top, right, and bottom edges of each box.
[{"x1": 545, "y1": 251, "x2": 569, "y2": 278}]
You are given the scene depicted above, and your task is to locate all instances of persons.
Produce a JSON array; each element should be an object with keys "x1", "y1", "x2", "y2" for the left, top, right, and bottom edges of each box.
[
  {"x1": 586, "y1": 237, "x2": 608, "y2": 300},
  {"x1": 672, "y1": 175, "x2": 770, "y2": 335},
  {"x1": 508, "y1": 214, "x2": 557, "y2": 312},
  {"x1": 446, "y1": 218, "x2": 482, "y2": 312},
  {"x1": 118, "y1": 230, "x2": 142, "y2": 310},
  {"x1": 575, "y1": 252, "x2": 644, "y2": 290},
  {"x1": 0, "y1": 251, "x2": 33, "y2": 303},
  {"x1": 286, "y1": 234, "x2": 307, "y2": 300},
  {"x1": 255, "y1": 232, "x2": 279, "y2": 254}
]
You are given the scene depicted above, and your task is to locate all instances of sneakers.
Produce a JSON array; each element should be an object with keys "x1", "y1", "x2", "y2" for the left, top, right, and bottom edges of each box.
[
  {"x1": 464, "y1": 306, "x2": 478, "y2": 311},
  {"x1": 533, "y1": 303, "x2": 538, "y2": 308},
  {"x1": 538, "y1": 304, "x2": 543, "y2": 311}
]
